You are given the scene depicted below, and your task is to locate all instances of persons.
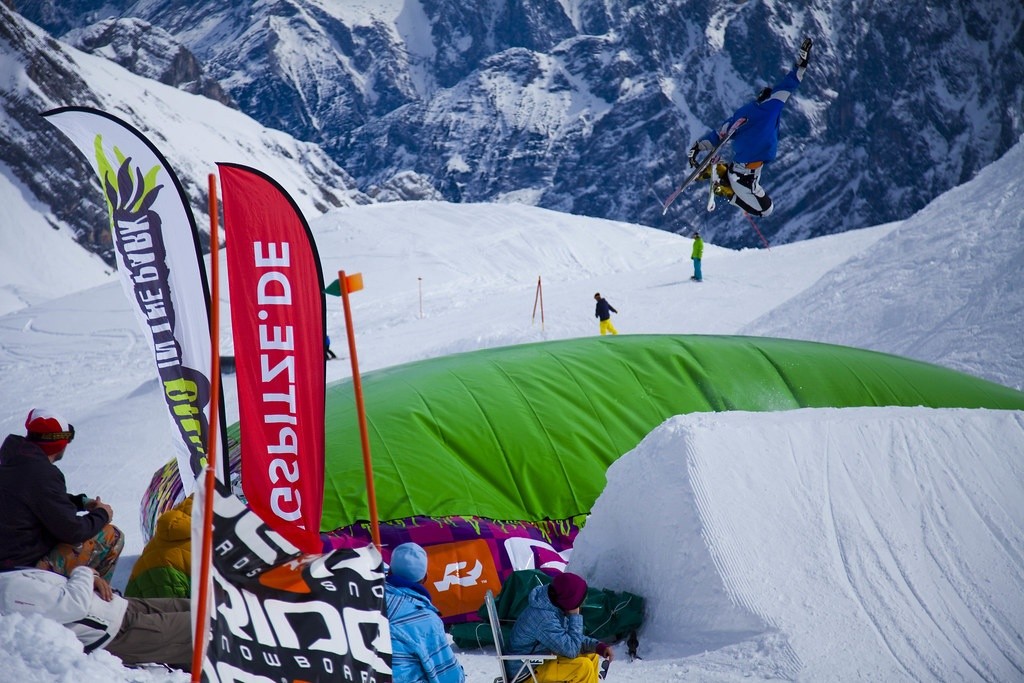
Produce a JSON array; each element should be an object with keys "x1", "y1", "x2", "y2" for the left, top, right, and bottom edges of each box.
[
  {"x1": 122, "y1": 494, "x2": 194, "y2": 599},
  {"x1": 0, "y1": 565, "x2": 193, "y2": 673},
  {"x1": 503, "y1": 572, "x2": 614, "y2": 683},
  {"x1": 384, "y1": 542, "x2": 466, "y2": 683},
  {"x1": 594, "y1": 292, "x2": 618, "y2": 336},
  {"x1": 326, "y1": 335, "x2": 336, "y2": 359},
  {"x1": 688, "y1": 37, "x2": 812, "y2": 218},
  {"x1": 0, "y1": 409, "x2": 125, "y2": 588},
  {"x1": 691, "y1": 232, "x2": 704, "y2": 282}
]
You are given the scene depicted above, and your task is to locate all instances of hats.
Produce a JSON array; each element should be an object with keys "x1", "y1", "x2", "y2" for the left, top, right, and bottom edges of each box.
[
  {"x1": 549, "y1": 572, "x2": 588, "y2": 612},
  {"x1": 391, "y1": 542, "x2": 428, "y2": 583},
  {"x1": 25, "y1": 408, "x2": 71, "y2": 456},
  {"x1": 757, "y1": 88, "x2": 772, "y2": 103},
  {"x1": 594, "y1": 293, "x2": 600, "y2": 299}
]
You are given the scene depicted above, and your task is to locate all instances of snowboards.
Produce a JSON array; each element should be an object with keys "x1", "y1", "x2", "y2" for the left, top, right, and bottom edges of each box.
[{"x1": 662, "y1": 116, "x2": 749, "y2": 216}]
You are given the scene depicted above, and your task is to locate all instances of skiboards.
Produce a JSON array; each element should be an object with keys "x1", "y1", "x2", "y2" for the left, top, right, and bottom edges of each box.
[{"x1": 327, "y1": 358, "x2": 346, "y2": 360}]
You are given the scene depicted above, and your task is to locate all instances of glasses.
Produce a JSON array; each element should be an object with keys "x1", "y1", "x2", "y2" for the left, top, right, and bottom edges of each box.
[
  {"x1": 598, "y1": 657, "x2": 610, "y2": 680},
  {"x1": 67, "y1": 424, "x2": 75, "y2": 442}
]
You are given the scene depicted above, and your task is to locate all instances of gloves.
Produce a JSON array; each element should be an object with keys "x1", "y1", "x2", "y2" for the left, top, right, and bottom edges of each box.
[
  {"x1": 797, "y1": 38, "x2": 813, "y2": 68},
  {"x1": 688, "y1": 142, "x2": 703, "y2": 169}
]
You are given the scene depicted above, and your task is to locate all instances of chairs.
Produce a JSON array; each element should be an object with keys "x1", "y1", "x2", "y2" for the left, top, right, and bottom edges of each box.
[{"x1": 485, "y1": 590, "x2": 558, "y2": 683}]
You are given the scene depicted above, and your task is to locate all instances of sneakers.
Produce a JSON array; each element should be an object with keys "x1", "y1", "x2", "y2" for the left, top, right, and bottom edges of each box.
[
  {"x1": 700, "y1": 163, "x2": 726, "y2": 182},
  {"x1": 713, "y1": 184, "x2": 734, "y2": 200}
]
